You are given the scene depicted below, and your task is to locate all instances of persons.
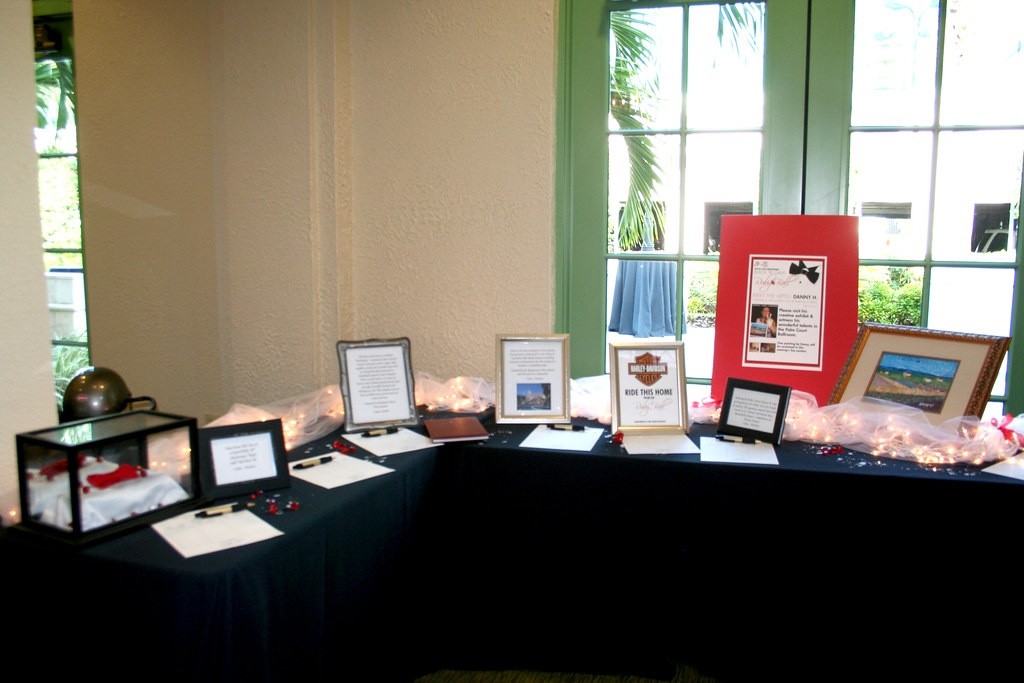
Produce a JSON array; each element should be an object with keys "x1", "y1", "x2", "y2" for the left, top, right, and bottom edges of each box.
[{"x1": 756, "y1": 307, "x2": 776, "y2": 340}]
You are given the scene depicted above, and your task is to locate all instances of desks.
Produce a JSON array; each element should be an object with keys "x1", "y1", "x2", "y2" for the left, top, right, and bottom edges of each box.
[
  {"x1": 0, "y1": 404, "x2": 488, "y2": 683},
  {"x1": 608, "y1": 252, "x2": 687, "y2": 338},
  {"x1": 449, "y1": 410, "x2": 1024, "y2": 683}
]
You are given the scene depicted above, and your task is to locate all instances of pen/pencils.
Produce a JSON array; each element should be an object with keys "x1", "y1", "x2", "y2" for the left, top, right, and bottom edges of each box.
[
  {"x1": 361, "y1": 427, "x2": 401, "y2": 439},
  {"x1": 546, "y1": 423, "x2": 590, "y2": 432},
  {"x1": 715, "y1": 434, "x2": 762, "y2": 444},
  {"x1": 292, "y1": 453, "x2": 337, "y2": 473},
  {"x1": 195, "y1": 502, "x2": 255, "y2": 523}
]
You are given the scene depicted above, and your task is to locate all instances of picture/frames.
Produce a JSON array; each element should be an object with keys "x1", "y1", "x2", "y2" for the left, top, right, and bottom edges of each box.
[
  {"x1": 827, "y1": 321, "x2": 1012, "y2": 440},
  {"x1": 335, "y1": 337, "x2": 421, "y2": 434},
  {"x1": 198, "y1": 418, "x2": 292, "y2": 503},
  {"x1": 495, "y1": 332, "x2": 571, "y2": 424},
  {"x1": 608, "y1": 342, "x2": 691, "y2": 435},
  {"x1": 716, "y1": 378, "x2": 792, "y2": 446}
]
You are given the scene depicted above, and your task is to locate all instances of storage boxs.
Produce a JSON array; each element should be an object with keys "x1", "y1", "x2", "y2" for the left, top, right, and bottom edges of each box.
[{"x1": 15, "y1": 409, "x2": 201, "y2": 551}]
